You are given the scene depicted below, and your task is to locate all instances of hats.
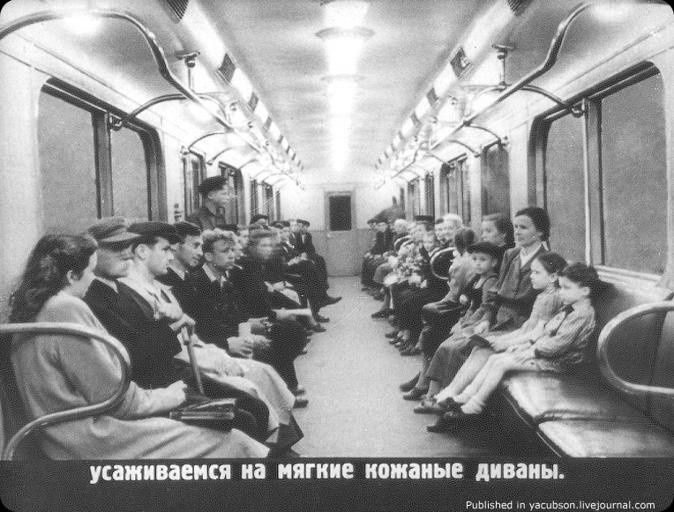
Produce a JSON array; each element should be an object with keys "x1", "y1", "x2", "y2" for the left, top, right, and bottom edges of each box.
[
  {"x1": 467, "y1": 242, "x2": 498, "y2": 255},
  {"x1": 81, "y1": 216, "x2": 141, "y2": 244},
  {"x1": 127, "y1": 223, "x2": 182, "y2": 244},
  {"x1": 197, "y1": 176, "x2": 226, "y2": 196}
]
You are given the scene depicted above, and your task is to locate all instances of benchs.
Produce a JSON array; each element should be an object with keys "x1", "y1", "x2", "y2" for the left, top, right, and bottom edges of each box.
[
  {"x1": 534, "y1": 296, "x2": 673, "y2": 461},
  {"x1": 500, "y1": 277, "x2": 673, "y2": 429}
]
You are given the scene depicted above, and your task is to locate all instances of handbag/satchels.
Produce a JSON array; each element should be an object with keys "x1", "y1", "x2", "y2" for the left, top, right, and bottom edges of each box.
[
  {"x1": 168, "y1": 396, "x2": 237, "y2": 432},
  {"x1": 421, "y1": 300, "x2": 464, "y2": 326}
]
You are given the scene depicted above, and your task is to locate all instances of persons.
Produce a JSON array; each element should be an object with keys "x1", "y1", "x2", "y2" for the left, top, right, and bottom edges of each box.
[
  {"x1": 4, "y1": 213, "x2": 341, "y2": 462},
  {"x1": 360, "y1": 206, "x2": 603, "y2": 434},
  {"x1": 187, "y1": 176, "x2": 231, "y2": 228}
]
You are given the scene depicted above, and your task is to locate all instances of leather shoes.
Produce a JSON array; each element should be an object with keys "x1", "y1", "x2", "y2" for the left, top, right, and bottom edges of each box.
[
  {"x1": 396, "y1": 338, "x2": 406, "y2": 346},
  {"x1": 400, "y1": 374, "x2": 418, "y2": 391},
  {"x1": 403, "y1": 387, "x2": 427, "y2": 399},
  {"x1": 290, "y1": 384, "x2": 304, "y2": 392},
  {"x1": 400, "y1": 344, "x2": 413, "y2": 354},
  {"x1": 327, "y1": 296, "x2": 341, "y2": 304},
  {"x1": 313, "y1": 323, "x2": 326, "y2": 331},
  {"x1": 389, "y1": 336, "x2": 400, "y2": 343},
  {"x1": 400, "y1": 343, "x2": 409, "y2": 350},
  {"x1": 294, "y1": 396, "x2": 307, "y2": 407},
  {"x1": 316, "y1": 313, "x2": 328, "y2": 322}
]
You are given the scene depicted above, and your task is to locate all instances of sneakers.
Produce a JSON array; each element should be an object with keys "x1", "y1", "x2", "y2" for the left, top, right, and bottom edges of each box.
[
  {"x1": 444, "y1": 405, "x2": 480, "y2": 421},
  {"x1": 422, "y1": 398, "x2": 442, "y2": 411},
  {"x1": 427, "y1": 417, "x2": 446, "y2": 432},
  {"x1": 385, "y1": 330, "x2": 397, "y2": 338},
  {"x1": 371, "y1": 311, "x2": 389, "y2": 318},
  {"x1": 414, "y1": 406, "x2": 430, "y2": 413},
  {"x1": 444, "y1": 397, "x2": 464, "y2": 409}
]
